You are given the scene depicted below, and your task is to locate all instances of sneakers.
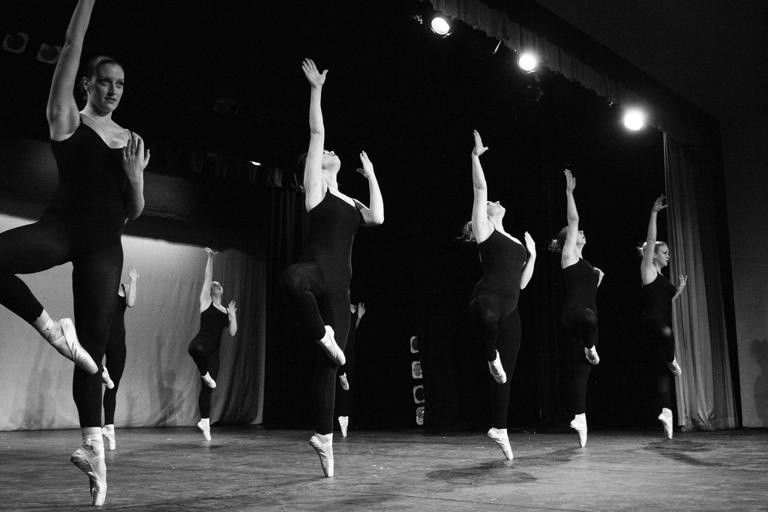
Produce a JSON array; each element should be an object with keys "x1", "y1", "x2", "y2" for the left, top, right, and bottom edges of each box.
[
  {"x1": 668, "y1": 355, "x2": 681, "y2": 376},
  {"x1": 584, "y1": 346, "x2": 600, "y2": 365},
  {"x1": 570, "y1": 419, "x2": 588, "y2": 447},
  {"x1": 70, "y1": 449, "x2": 107, "y2": 506},
  {"x1": 340, "y1": 372, "x2": 350, "y2": 390},
  {"x1": 102, "y1": 426, "x2": 116, "y2": 450},
  {"x1": 203, "y1": 372, "x2": 216, "y2": 389},
  {"x1": 310, "y1": 435, "x2": 335, "y2": 477},
  {"x1": 197, "y1": 421, "x2": 211, "y2": 441},
  {"x1": 325, "y1": 325, "x2": 346, "y2": 366},
  {"x1": 101, "y1": 366, "x2": 114, "y2": 389},
  {"x1": 658, "y1": 413, "x2": 673, "y2": 440},
  {"x1": 487, "y1": 427, "x2": 513, "y2": 459},
  {"x1": 338, "y1": 416, "x2": 348, "y2": 437},
  {"x1": 487, "y1": 351, "x2": 506, "y2": 385},
  {"x1": 53, "y1": 318, "x2": 99, "y2": 375}
]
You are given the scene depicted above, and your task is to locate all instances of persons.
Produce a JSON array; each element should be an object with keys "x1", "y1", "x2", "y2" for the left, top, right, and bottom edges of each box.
[
  {"x1": 0, "y1": 0, "x2": 153, "y2": 508},
  {"x1": 104, "y1": 270, "x2": 139, "y2": 449},
  {"x1": 557, "y1": 168, "x2": 604, "y2": 448},
  {"x1": 295, "y1": 58, "x2": 384, "y2": 477},
  {"x1": 189, "y1": 247, "x2": 238, "y2": 442},
  {"x1": 461, "y1": 129, "x2": 537, "y2": 461},
  {"x1": 638, "y1": 196, "x2": 690, "y2": 439}
]
